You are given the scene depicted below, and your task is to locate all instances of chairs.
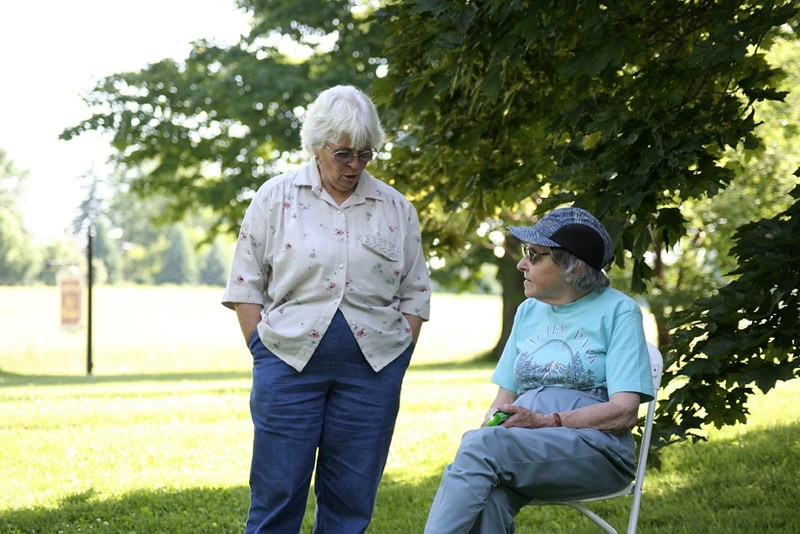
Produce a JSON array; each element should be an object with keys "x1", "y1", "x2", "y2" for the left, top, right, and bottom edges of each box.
[{"x1": 526, "y1": 340, "x2": 663, "y2": 534}]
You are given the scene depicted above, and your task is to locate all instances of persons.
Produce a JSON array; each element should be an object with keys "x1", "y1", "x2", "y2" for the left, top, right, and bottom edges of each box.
[
  {"x1": 425, "y1": 207, "x2": 655, "y2": 534},
  {"x1": 223, "y1": 85, "x2": 431, "y2": 534}
]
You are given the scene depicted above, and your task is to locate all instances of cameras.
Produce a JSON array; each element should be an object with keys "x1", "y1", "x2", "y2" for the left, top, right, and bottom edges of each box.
[{"x1": 488, "y1": 412, "x2": 511, "y2": 427}]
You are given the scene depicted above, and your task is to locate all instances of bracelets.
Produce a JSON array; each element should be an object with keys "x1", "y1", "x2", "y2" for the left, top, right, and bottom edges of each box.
[{"x1": 552, "y1": 410, "x2": 562, "y2": 429}]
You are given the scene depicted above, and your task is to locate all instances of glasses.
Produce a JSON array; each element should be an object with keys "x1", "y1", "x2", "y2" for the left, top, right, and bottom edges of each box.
[
  {"x1": 323, "y1": 144, "x2": 372, "y2": 163},
  {"x1": 521, "y1": 244, "x2": 551, "y2": 264}
]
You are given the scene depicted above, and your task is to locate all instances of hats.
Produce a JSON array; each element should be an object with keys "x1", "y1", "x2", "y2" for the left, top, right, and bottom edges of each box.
[{"x1": 504, "y1": 207, "x2": 615, "y2": 271}]
